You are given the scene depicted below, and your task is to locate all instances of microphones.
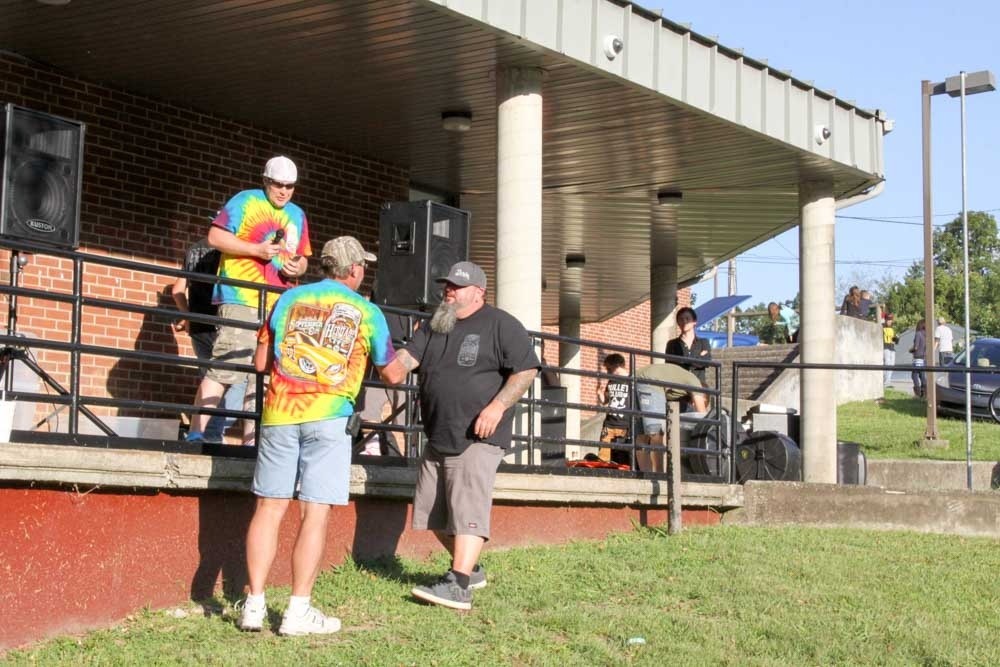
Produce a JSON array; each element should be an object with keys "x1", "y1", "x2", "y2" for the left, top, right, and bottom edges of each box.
[{"x1": 272, "y1": 229, "x2": 284, "y2": 244}]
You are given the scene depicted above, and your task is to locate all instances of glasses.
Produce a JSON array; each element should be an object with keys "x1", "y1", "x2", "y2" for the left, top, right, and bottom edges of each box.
[
  {"x1": 268, "y1": 179, "x2": 295, "y2": 189},
  {"x1": 358, "y1": 262, "x2": 368, "y2": 269}
]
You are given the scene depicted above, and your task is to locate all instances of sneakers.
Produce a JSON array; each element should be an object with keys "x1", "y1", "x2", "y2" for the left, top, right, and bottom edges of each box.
[
  {"x1": 278, "y1": 607, "x2": 342, "y2": 636},
  {"x1": 240, "y1": 602, "x2": 268, "y2": 631},
  {"x1": 412, "y1": 571, "x2": 472, "y2": 610},
  {"x1": 430, "y1": 565, "x2": 487, "y2": 591}
]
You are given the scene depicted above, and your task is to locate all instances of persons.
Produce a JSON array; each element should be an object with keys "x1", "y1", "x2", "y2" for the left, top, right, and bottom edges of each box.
[
  {"x1": 584, "y1": 307, "x2": 712, "y2": 472},
  {"x1": 840, "y1": 285, "x2": 900, "y2": 388},
  {"x1": 238, "y1": 236, "x2": 411, "y2": 635},
  {"x1": 540, "y1": 337, "x2": 561, "y2": 388},
  {"x1": 909, "y1": 310, "x2": 953, "y2": 399},
  {"x1": 768, "y1": 301, "x2": 800, "y2": 343},
  {"x1": 173, "y1": 155, "x2": 313, "y2": 446},
  {"x1": 379, "y1": 261, "x2": 542, "y2": 610}
]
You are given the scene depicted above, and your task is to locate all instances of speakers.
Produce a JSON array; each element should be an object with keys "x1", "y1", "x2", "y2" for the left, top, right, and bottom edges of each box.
[
  {"x1": 372, "y1": 200, "x2": 472, "y2": 312},
  {"x1": 0, "y1": 102, "x2": 86, "y2": 254}
]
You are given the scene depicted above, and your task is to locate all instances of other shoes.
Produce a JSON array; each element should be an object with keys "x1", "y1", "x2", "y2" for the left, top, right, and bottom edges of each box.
[{"x1": 185, "y1": 432, "x2": 204, "y2": 442}]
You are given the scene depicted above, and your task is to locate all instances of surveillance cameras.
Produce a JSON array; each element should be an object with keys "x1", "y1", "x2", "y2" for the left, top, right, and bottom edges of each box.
[
  {"x1": 814, "y1": 125, "x2": 831, "y2": 145},
  {"x1": 603, "y1": 35, "x2": 624, "y2": 59}
]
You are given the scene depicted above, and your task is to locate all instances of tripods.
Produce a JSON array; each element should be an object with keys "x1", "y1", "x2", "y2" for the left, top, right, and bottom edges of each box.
[{"x1": 0, "y1": 251, "x2": 119, "y2": 438}]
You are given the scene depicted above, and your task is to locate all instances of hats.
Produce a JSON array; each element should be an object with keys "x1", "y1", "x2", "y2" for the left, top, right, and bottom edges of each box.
[
  {"x1": 262, "y1": 155, "x2": 298, "y2": 183},
  {"x1": 939, "y1": 317, "x2": 944, "y2": 322},
  {"x1": 435, "y1": 262, "x2": 487, "y2": 289},
  {"x1": 321, "y1": 236, "x2": 377, "y2": 266}
]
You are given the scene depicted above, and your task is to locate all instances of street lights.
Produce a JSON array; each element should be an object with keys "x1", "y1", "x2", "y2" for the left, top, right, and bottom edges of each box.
[{"x1": 922, "y1": 69, "x2": 995, "y2": 442}]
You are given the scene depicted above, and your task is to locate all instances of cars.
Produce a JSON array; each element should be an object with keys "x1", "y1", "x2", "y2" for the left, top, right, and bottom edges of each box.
[
  {"x1": 692, "y1": 295, "x2": 759, "y2": 348},
  {"x1": 935, "y1": 337, "x2": 1000, "y2": 424}
]
